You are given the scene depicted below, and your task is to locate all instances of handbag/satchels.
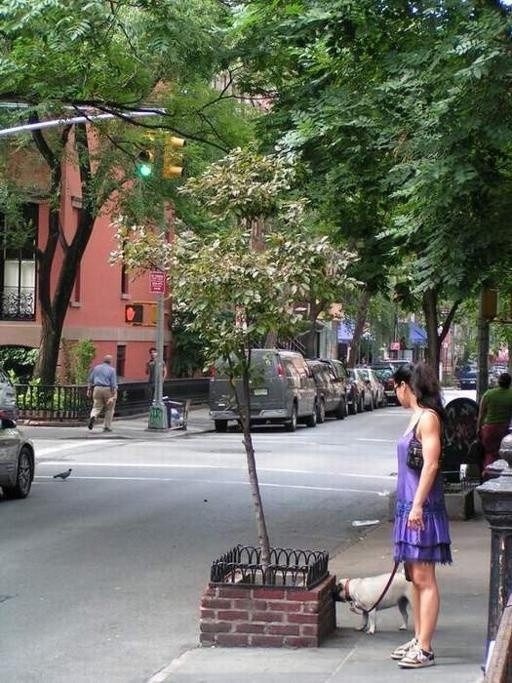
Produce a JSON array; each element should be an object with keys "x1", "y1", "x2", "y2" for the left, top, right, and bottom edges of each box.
[{"x1": 406, "y1": 410, "x2": 447, "y2": 473}]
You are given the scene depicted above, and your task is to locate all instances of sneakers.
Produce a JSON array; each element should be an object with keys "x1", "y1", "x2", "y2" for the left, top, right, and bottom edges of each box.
[
  {"x1": 397, "y1": 644, "x2": 435, "y2": 668},
  {"x1": 88, "y1": 416, "x2": 96, "y2": 430},
  {"x1": 390, "y1": 637, "x2": 419, "y2": 660},
  {"x1": 103, "y1": 427, "x2": 112, "y2": 432}
]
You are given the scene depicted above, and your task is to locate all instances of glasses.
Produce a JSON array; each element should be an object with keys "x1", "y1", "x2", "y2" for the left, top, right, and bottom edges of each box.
[{"x1": 394, "y1": 385, "x2": 400, "y2": 394}]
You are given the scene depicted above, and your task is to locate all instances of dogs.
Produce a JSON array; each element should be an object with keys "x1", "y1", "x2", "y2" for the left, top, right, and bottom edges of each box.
[{"x1": 334, "y1": 569, "x2": 413, "y2": 634}]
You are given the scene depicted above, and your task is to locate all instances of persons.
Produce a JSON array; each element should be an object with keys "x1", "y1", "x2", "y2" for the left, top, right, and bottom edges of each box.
[
  {"x1": 474, "y1": 370, "x2": 512, "y2": 483},
  {"x1": 145, "y1": 347, "x2": 168, "y2": 401},
  {"x1": 86, "y1": 354, "x2": 118, "y2": 432},
  {"x1": 384, "y1": 360, "x2": 451, "y2": 669}
]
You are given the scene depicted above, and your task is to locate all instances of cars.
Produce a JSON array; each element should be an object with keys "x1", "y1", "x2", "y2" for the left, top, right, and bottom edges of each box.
[
  {"x1": 208, "y1": 348, "x2": 410, "y2": 432},
  {"x1": 0, "y1": 365, "x2": 35, "y2": 499},
  {"x1": 458, "y1": 364, "x2": 508, "y2": 390}
]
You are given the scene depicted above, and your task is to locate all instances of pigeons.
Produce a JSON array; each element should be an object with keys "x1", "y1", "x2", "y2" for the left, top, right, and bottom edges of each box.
[{"x1": 53, "y1": 468, "x2": 72, "y2": 480}]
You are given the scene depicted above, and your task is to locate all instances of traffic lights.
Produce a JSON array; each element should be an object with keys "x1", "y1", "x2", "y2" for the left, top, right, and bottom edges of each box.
[
  {"x1": 138, "y1": 136, "x2": 155, "y2": 179},
  {"x1": 170, "y1": 138, "x2": 187, "y2": 177},
  {"x1": 123, "y1": 301, "x2": 157, "y2": 329}
]
